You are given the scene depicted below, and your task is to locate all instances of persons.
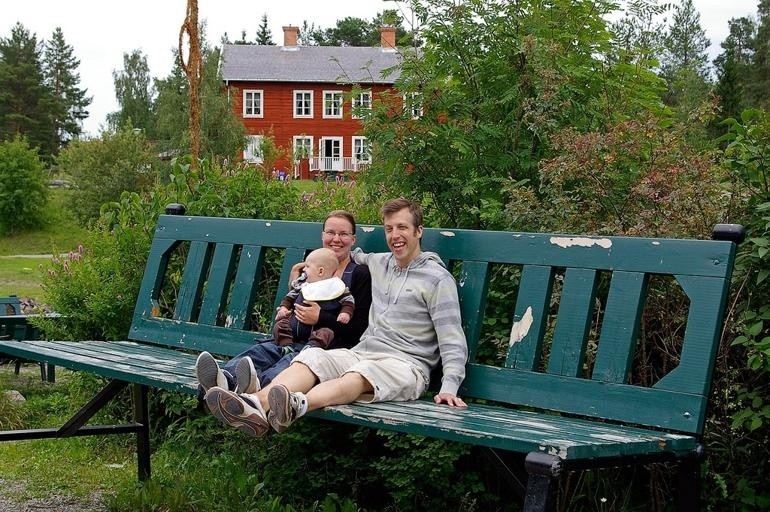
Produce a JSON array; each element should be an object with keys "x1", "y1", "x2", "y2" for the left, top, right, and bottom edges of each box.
[
  {"x1": 196, "y1": 210, "x2": 372, "y2": 394},
  {"x1": 270, "y1": 166, "x2": 290, "y2": 182},
  {"x1": 204, "y1": 199, "x2": 468, "y2": 438},
  {"x1": 272, "y1": 248, "x2": 354, "y2": 353}
]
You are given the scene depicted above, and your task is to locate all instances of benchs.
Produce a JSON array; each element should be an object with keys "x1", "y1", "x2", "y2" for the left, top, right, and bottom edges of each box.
[{"x1": 2, "y1": 202, "x2": 745, "y2": 510}]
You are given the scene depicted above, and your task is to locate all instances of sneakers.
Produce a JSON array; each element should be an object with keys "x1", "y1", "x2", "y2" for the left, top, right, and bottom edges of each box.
[
  {"x1": 282, "y1": 346, "x2": 294, "y2": 355},
  {"x1": 195, "y1": 351, "x2": 304, "y2": 438}
]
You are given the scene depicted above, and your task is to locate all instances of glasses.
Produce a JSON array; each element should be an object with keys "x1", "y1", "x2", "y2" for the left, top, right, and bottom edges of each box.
[{"x1": 323, "y1": 230, "x2": 352, "y2": 238}]
